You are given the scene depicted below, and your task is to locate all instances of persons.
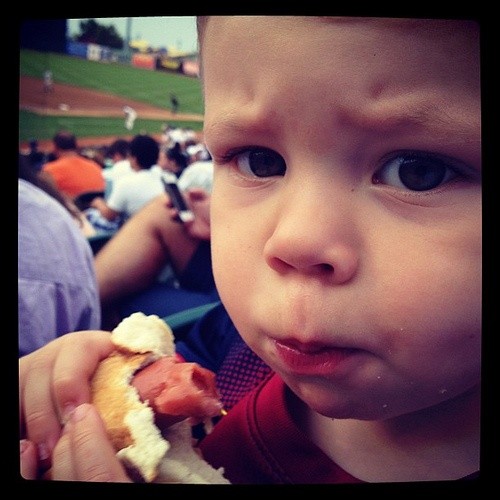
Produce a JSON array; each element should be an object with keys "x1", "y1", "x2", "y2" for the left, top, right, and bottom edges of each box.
[
  {"x1": 19, "y1": 16, "x2": 484, "y2": 485},
  {"x1": 90, "y1": 187, "x2": 219, "y2": 306},
  {"x1": 170, "y1": 91, "x2": 184, "y2": 118},
  {"x1": 19, "y1": 122, "x2": 216, "y2": 234},
  {"x1": 17, "y1": 176, "x2": 104, "y2": 359}
]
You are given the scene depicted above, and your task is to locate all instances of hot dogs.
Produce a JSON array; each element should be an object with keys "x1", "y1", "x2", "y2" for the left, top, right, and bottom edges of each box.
[{"x1": 83, "y1": 312, "x2": 234, "y2": 484}]
